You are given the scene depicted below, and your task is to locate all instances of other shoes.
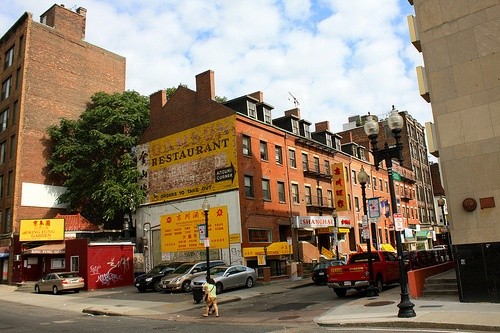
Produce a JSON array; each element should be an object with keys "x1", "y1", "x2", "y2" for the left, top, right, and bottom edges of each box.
[
  {"x1": 215, "y1": 314, "x2": 219, "y2": 317},
  {"x1": 203, "y1": 314, "x2": 209, "y2": 317}
]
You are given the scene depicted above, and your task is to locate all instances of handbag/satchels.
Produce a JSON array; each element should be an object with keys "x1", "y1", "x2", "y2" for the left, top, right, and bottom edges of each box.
[{"x1": 205, "y1": 293, "x2": 208, "y2": 301}]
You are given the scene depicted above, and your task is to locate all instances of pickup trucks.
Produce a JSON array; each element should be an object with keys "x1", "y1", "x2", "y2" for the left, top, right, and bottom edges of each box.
[{"x1": 328, "y1": 250, "x2": 401, "y2": 298}]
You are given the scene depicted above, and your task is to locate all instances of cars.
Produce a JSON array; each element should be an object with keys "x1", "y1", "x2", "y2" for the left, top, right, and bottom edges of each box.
[
  {"x1": 134, "y1": 258, "x2": 258, "y2": 294},
  {"x1": 34, "y1": 272, "x2": 85, "y2": 294},
  {"x1": 395, "y1": 244, "x2": 450, "y2": 281},
  {"x1": 312, "y1": 261, "x2": 345, "y2": 285}
]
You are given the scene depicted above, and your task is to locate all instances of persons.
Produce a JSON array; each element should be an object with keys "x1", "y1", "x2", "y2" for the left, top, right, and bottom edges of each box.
[
  {"x1": 201, "y1": 276, "x2": 215, "y2": 315},
  {"x1": 202, "y1": 279, "x2": 220, "y2": 317}
]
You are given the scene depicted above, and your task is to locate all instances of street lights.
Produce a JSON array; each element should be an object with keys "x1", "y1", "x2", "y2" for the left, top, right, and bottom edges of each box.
[
  {"x1": 437, "y1": 192, "x2": 447, "y2": 227},
  {"x1": 202, "y1": 196, "x2": 210, "y2": 283},
  {"x1": 364, "y1": 103, "x2": 416, "y2": 317},
  {"x1": 357, "y1": 165, "x2": 379, "y2": 296}
]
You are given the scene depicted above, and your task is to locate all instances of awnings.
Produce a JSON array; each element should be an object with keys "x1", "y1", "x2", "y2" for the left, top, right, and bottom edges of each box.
[{"x1": 27, "y1": 245, "x2": 66, "y2": 254}]
